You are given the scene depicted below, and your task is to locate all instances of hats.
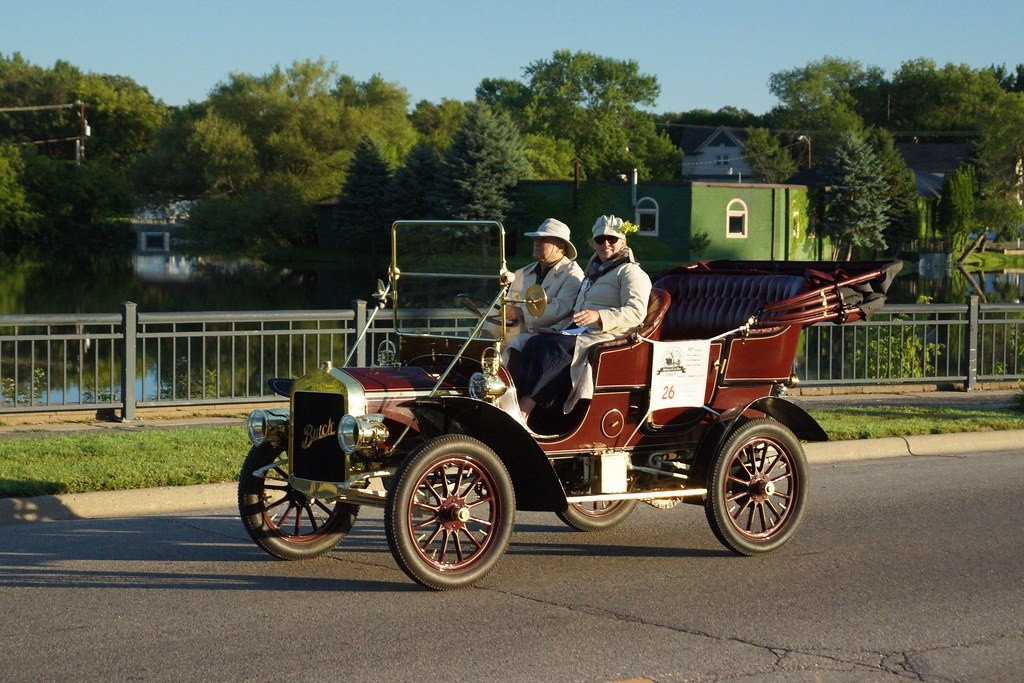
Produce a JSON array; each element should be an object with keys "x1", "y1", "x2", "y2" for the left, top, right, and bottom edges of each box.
[
  {"x1": 523, "y1": 217, "x2": 578, "y2": 260},
  {"x1": 591, "y1": 214, "x2": 626, "y2": 239}
]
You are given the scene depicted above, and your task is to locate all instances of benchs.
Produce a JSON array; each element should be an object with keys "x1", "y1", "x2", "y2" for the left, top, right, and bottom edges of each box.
[{"x1": 654, "y1": 274, "x2": 804, "y2": 346}]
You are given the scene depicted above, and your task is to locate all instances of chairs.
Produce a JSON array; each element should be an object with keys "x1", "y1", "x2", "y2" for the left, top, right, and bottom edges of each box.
[{"x1": 544, "y1": 286, "x2": 672, "y2": 394}]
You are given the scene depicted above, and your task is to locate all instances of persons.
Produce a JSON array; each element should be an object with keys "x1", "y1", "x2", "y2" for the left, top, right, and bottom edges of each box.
[
  {"x1": 502, "y1": 215, "x2": 652, "y2": 426},
  {"x1": 478, "y1": 218, "x2": 585, "y2": 402}
]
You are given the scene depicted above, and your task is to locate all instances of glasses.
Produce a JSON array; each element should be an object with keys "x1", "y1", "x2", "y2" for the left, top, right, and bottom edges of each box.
[{"x1": 594, "y1": 235, "x2": 620, "y2": 244}]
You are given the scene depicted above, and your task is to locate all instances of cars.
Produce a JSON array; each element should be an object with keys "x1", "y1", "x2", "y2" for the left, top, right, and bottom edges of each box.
[{"x1": 238, "y1": 219, "x2": 903, "y2": 593}]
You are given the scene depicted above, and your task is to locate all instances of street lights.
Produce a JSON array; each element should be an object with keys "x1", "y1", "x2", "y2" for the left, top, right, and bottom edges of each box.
[{"x1": 797, "y1": 135, "x2": 812, "y2": 169}]
